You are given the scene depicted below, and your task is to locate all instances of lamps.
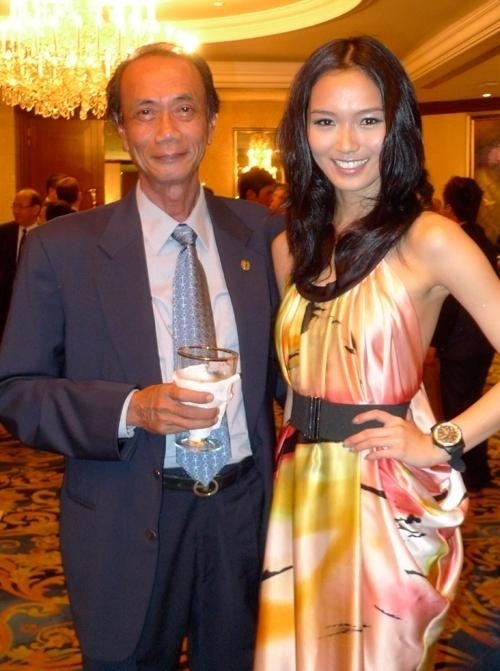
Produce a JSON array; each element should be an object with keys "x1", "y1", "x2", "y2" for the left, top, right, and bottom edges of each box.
[{"x1": 0, "y1": 0, "x2": 161, "y2": 120}]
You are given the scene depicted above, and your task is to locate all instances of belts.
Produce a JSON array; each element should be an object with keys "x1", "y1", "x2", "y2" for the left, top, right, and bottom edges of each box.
[{"x1": 162, "y1": 460, "x2": 251, "y2": 497}]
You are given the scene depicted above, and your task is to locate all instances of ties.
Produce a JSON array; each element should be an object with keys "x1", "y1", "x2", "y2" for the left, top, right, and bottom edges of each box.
[
  {"x1": 172, "y1": 224, "x2": 231, "y2": 486},
  {"x1": 17, "y1": 229, "x2": 27, "y2": 258}
]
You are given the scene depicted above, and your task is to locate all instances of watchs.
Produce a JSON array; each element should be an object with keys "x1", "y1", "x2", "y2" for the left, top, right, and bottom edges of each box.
[{"x1": 430, "y1": 421, "x2": 466, "y2": 462}]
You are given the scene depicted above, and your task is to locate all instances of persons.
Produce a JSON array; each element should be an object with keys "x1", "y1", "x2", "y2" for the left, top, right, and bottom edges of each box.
[
  {"x1": 238, "y1": 165, "x2": 288, "y2": 215},
  {"x1": 252, "y1": 35, "x2": 500, "y2": 670},
  {"x1": 430, "y1": 175, "x2": 497, "y2": 492},
  {"x1": 0, "y1": 42, "x2": 289, "y2": 671},
  {"x1": 0, "y1": 174, "x2": 82, "y2": 336}
]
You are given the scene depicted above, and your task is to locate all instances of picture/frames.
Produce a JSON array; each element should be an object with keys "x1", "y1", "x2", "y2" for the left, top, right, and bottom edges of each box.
[
  {"x1": 232, "y1": 127, "x2": 286, "y2": 199},
  {"x1": 464, "y1": 110, "x2": 500, "y2": 261}
]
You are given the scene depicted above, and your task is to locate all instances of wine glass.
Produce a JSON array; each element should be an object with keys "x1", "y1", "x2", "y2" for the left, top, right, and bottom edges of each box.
[{"x1": 171, "y1": 344, "x2": 240, "y2": 454}]
